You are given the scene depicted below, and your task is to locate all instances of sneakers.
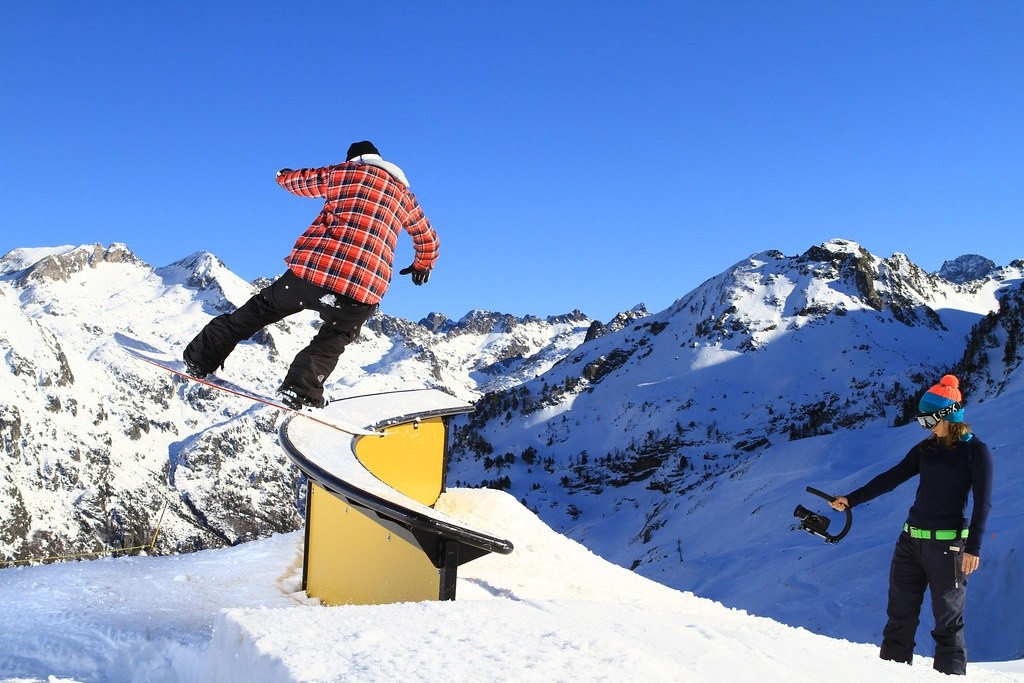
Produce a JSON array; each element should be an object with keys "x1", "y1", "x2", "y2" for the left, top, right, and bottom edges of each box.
[
  {"x1": 182, "y1": 358, "x2": 209, "y2": 380},
  {"x1": 275, "y1": 389, "x2": 330, "y2": 411}
]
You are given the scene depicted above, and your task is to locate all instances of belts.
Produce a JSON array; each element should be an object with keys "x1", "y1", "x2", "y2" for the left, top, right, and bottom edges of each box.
[{"x1": 903, "y1": 522, "x2": 970, "y2": 540}]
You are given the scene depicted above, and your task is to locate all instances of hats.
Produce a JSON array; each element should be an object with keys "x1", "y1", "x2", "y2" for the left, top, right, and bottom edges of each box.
[
  {"x1": 345, "y1": 140, "x2": 381, "y2": 162},
  {"x1": 919, "y1": 374, "x2": 965, "y2": 423}
]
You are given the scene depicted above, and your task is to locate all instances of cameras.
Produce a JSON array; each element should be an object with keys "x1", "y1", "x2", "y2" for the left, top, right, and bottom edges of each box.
[{"x1": 794, "y1": 505, "x2": 831, "y2": 531}]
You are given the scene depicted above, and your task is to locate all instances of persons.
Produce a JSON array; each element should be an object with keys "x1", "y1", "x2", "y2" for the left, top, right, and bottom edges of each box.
[
  {"x1": 182, "y1": 142, "x2": 439, "y2": 408},
  {"x1": 827, "y1": 376, "x2": 992, "y2": 676}
]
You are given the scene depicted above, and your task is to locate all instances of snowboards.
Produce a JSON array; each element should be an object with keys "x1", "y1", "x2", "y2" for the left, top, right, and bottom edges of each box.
[{"x1": 114, "y1": 331, "x2": 393, "y2": 437}]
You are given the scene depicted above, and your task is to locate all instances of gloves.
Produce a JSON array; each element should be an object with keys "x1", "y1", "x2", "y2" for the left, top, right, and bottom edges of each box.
[{"x1": 399, "y1": 263, "x2": 431, "y2": 286}]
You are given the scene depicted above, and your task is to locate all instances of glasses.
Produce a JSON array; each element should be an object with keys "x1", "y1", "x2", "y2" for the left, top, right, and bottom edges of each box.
[{"x1": 915, "y1": 401, "x2": 963, "y2": 430}]
